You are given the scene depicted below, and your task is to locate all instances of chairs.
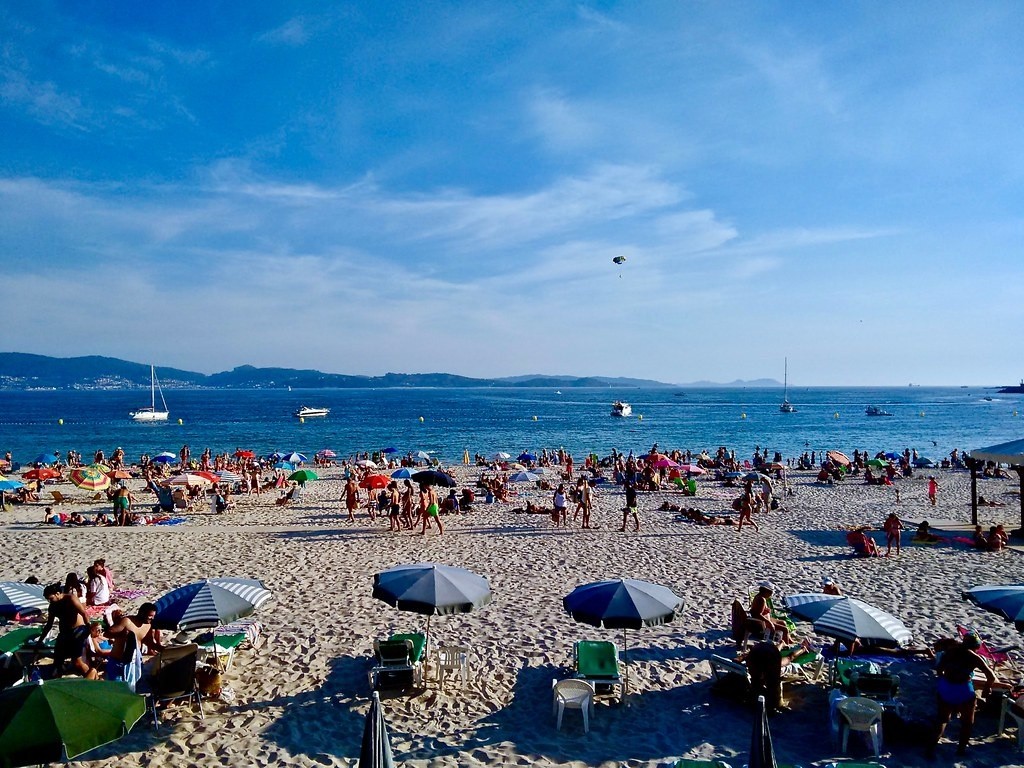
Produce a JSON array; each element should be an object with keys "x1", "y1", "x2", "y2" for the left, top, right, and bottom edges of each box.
[
  {"x1": 0, "y1": 617, "x2": 262, "y2": 730},
  {"x1": 971, "y1": 655, "x2": 1011, "y2": 693},
  {"x1": 553, "y1": 678, "x2": 595, "y2": 733},
  {"x1": 995, "y1": 695, "x2": 1024, "y2": 751},
  {"x1": 436, "y1": 645, "x2": 470, "y2": 692},
  {"x1": 675, "y1": 587, "x2": 900, "y2": 768},
  {"x1": 11, "y1": 462, "x2": 457, "y2": 517},
  {"x1": 573, "y1": 638, "x2": 626, "y2": 706},
  {"x1": 368, "y1": 635, "x2": 427, "y2": 690}
]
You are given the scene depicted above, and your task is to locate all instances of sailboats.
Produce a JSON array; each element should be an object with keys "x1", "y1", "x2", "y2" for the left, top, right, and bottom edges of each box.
[
  {"x1": 128, "y1": 365, "x2": 170, "y2": 423},
  {"x1": 780, "y1": 357, "x2": 797, "y2": 412}
]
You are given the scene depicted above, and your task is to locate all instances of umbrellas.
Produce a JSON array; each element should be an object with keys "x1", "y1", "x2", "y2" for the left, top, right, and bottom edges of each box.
[
  {"x1": 106, "y1": 471, "x2": 132, "y2": 478},
  {"x1": 830, "y1": 449, "x2": 849, "y2": 466},
  {"x1": 653, "y1": 460, "x2": 680, "y2": 467},
  {"x1": 0, "y1": 481, "x2": 24, "y2": 489},
  {"x1": 783, "y1": 592, "x2": 914, "y2": 647},
  {"x1": 961, "y1": 582, "x2": 1024, "y2": 632},
  {"x1": 356, "y1": 460, "x2": 377, "y2": 468},
  {"x1": 69, "y1": 467, "x2": 111, "y2": 490},
  {"x1": 679, "y1": 465, "x2": 706, "y2": 473},
  {"x1": 885, "y1": 451, "x2": 904, "y2": 459},
  {"x1": 756, "y1": 462, "x2": 785, "y2": 469},
  {"x1": 22, "y1": 469, "x2": 60, "y2": 480},
  {"x1": 289, "y1": 470, "x2": 318, "y2": 481},
  {"x1": 160, "y1": 473, "x2": 210, "y2": 485},
  {"x1": 563, "y1": 579, "x2": 684, "y2": 690},
  {"x1": 391, "y1": 468, "x2": 417, "y2": 478},
  {"x1": 646, "y1": 454, "x2": 669, "y2": 462},
  {"x1": 216, "y1": 469, "x2": 241, "y2": 482},
  {"x1": 383, "y1": 448, "x2": 397, "y2": 452},
  {"x1": 152, "y1": 456, "x2": 175, "y2": 463},
  {"x1": 282, "y1": 452, "x2": 308, "y2": 461},
  {"x1": 151, "y1": 577, "x2": 272, "y2": 666},
  {"x1": 509, "y1": 472, "x2": 540, "y2": 481},
  {"x1": 359, "y1": 475, "x2": 392, "y2": 489},
  {"x1": 865, "y1": 459, "x2": 889, "y2": 466},
  {"x1": 490, "y1": 451, "x2": 511, "y2": 459},
  {"x1": 233, "y1": 452, "x2": 254, "y2": 457},
  {"x1": 155, "y1": 452, "x2": 176, "y2": 458},
  {"x1": 267, "y1": 453, "x2": 285, "y2": 459},
  {"x1": 0, "y1": 581, "x2": 50, "y2": 616},
  {"x1": 193, "y1": 471, "x2": 221, "y2": 482},
  {"x1": 372, "y1": 562, "x2": 492, "y2": 687},
  {"x1": 411, "y1": 471, "x2": 457, "y2": 488},
  {"x1": 516, "y1": 454, "x2": 537, "y2": 461},
  {"x1": 319, "y1": 449, "x2": 336, "y2": 457}
]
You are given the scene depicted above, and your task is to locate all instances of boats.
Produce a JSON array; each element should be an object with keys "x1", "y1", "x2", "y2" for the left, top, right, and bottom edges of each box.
[
  {"x1": 292, "y1": 404, "x2": 331, "y2": 417},
  {"x1": 864, "y1": 405, "x2": 894, "y2": 416},
  {"x1": 984, "y1": 396, "x2": 992, "y2": 401},
  {"x1": 610, "y1": 399, "x2": 632, "y2": 416}
]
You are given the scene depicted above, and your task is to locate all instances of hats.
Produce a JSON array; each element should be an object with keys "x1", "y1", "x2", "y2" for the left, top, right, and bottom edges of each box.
[
  {"x1": 758, "y1": 581, "x2": 774, "y2": 593},
  {"x1": 823, "y1": 576, "x2": 834, "y2": 587},
  {"x1": 963, "y1": 632, "x2": 982, "y2": 649}
]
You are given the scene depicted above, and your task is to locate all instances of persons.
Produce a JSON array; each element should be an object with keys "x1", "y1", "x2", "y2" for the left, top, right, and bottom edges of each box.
[
  {"x1": 36, "y1": 558, "x2": 188, "y2": 682},
  {"x1": 0, "y1": 447, "x2": 1008, "y2": 559},
  {"x1": 735, "y1": 577, "x2": 840, "y2": 672},
  {"x1": 927, "y1": 631, "x2": 995, "y2": 759}
]
